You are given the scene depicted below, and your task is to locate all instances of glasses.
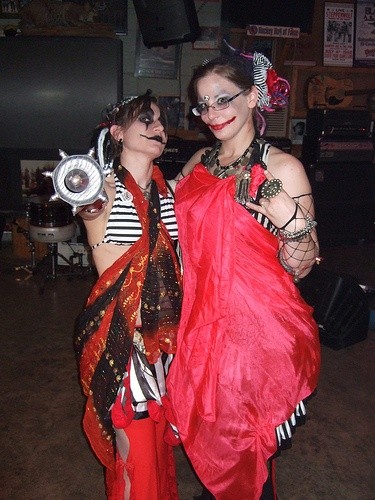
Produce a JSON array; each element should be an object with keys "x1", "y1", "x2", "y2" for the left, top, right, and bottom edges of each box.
[{"x1": 191, "y1": 88, "x2": 248, "y2": 116}]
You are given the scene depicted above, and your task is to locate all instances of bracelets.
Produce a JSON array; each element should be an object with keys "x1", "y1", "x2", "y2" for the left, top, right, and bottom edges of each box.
[{"x1": 278, "y1": 216, "x2": 315, "y2": 241}]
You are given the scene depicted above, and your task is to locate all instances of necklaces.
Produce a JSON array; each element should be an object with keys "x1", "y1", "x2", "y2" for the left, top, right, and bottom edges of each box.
[
  {"x1": 136, "y1": 180, "x2": 153, "y2": 190},
  {"x1": 213, "y1": 129, "x2": 261, "y2": 178}
]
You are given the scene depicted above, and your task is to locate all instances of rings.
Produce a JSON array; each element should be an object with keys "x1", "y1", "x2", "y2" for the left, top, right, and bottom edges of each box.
[{"x1": 261, "y1": 179, "x2": 283, "y2": 199}]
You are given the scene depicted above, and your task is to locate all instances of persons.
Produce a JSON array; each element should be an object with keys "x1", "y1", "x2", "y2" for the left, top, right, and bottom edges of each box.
[
  {"x1": 330, "y1": 21, "x2": 349, "y2": 41},
  {"x1": 21, "y1": 0, "x2": 97, "y2": 33},
  {"x1": 72, "y1": 94, "x2": 184, "y2": 500},
  {"x1": 291, "y1": 122, "x2": 306, "y2": 141},
  {"x1": 165, "y1": 54, "x2": 321, "y2": 500}
]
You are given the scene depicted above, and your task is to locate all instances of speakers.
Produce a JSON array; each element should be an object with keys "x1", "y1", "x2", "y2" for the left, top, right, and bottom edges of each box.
[
  {"x1": 301, "y1": 136, "x2": 375, "y2": 244},
  {"x1": 132, "y1": 0, "x2": 202, "y2": 49}
]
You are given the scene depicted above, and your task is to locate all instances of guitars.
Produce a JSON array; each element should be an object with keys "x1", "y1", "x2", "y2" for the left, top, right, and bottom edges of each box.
[{"x1": 304, "y1": 75, "x2": 375, "y2": 108}]
